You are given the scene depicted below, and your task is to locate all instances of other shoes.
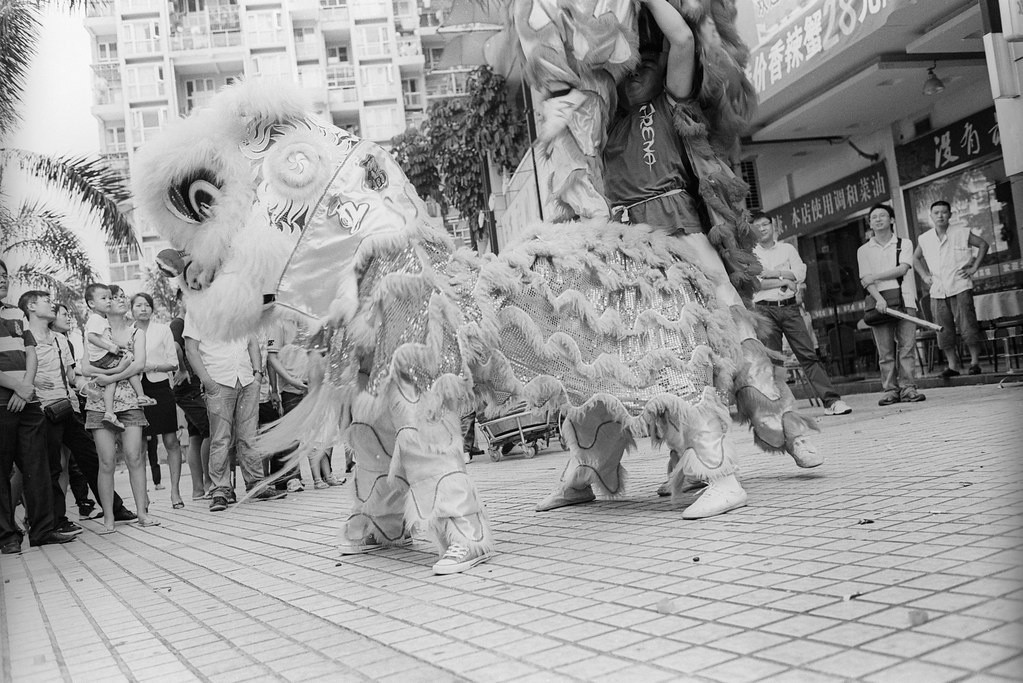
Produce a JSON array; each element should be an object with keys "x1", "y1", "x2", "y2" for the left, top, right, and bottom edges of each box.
[
  {"x1": 1, "y1": 460, "x2": 355, "y2": 555},
  {"x1": 969, "y1": 365, "x2": 982, "y2": 374},
  {"x1": 937, "y1": 368, "x2": 961, "y2": 378},
  {"x1": 463, "y1": 452, "x2": 471, "y2": 464},
  {"x1": 472, "y1": 449, "x2": 484, "y2": 455}
]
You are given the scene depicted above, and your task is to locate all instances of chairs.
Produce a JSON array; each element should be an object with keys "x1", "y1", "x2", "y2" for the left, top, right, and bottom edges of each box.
[
  {"x1": 909, "y1": 296, "x2": 1022, "y2": 373},
  {"x1": 826, "y1": 325, "x2": 861, "y2": 377}
]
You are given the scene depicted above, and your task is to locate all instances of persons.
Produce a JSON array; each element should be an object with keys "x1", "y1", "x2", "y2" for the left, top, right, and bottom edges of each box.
[
  {"x1": 601, "y1": 0, "x2": 823, "y2": 496},
  {"x1": 168, "y1": 285, "x2": 356, "y2": 511},
  {"x1": 856, "y1": 203, "x2": 926, "y2": 406},
  {"x1": 8, "y1": 303, "x2": 104, "y2": 530},
  {"x1": 460, "y1": 411, "x2": 547, "y2": 464},
  {"x1": 146, "y1": 435, "x2": 165, "y2": 490},
  {"x1": 178, "y1": 424, "x2": 189, "y2": 463},
  {"x1": 913, "y1": 200, "x2": 990, "y2": 378},
  {"x1": 84, "y1": 283, "x2": 157, "y2": 431},
  {"x1": 749, "y1": 212, "x2": 852, "y2": 415},
  {"x1": 17, "y1": 290, "x2": 138, "y2": 536},
  {"x1": 0, "y1": 260, "x2": 76, "y2": 553},
  {"x1": 81, "y1": 285, "x2": 162, "y2": 535},
  {"x1": 130, "y1": 293, "x2": 184, "y2": 511}
]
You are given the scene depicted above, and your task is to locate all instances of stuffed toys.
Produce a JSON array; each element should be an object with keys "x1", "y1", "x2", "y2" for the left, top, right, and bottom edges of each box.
[
  {"x1": 127, "y1": 71, "x2": 780, "y2": 575},
  {"x1": 507, "y1": 0, "x2": 763, "y2": 302}
]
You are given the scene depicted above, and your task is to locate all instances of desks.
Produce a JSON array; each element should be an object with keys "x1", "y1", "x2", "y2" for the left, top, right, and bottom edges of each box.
[{"x1": 915, "y1": 289, "x2": 1023, "y2": 372}]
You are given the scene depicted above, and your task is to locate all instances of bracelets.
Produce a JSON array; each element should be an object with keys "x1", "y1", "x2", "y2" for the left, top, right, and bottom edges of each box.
[
  {"x1": 154, "y1": 364, "x2": 158, "y2": 372},
  {"x1": 253, "y1": 370, "x2": 264, "y2": 377},
  {"x1": 778, "y1": 270, "x2": 783, "y2": 280}
]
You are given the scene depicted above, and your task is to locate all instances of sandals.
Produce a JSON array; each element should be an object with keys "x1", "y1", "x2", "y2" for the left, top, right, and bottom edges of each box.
[
  {"x1": 101, "y1": 411, "x2": 125, "y2": 432},
  {"x1": 138, "y1": 395, "x2": 157, "y2": 406}
]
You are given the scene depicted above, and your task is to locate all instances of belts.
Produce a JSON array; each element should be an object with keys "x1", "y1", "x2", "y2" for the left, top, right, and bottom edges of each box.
[{"x1": 755, "y1": 296, "x2": 796, "y2": 306}]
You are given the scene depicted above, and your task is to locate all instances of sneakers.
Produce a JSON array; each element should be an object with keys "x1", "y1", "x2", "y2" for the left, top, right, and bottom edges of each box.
[
  {"x1": 535, "y1": 484, "x2": 596, "y2": 512},
  {"x1": 900, "y1": 386, "x2": 926, "y2": 401},
  {"x1": 682, "y1": 481, "x2": 747, "y2": 519},
  {"x1": 824, "y1": 400, "x2": 852, "y2": 415},
  {"x1": 338, "y1": 528, "x2": 413, "y2": 554},
  {"x1": 432, "y1": 542, "x2": 492, "y2": 574},
  {"x1": 878, "y1": 390, "x2": 901, "y2": 405},
  {"x1": 787, "y1": 435, "x2": 824, "y2": 467},
  {"x1": 657, "y1": 475, "x2": 706, "y2": 495}
]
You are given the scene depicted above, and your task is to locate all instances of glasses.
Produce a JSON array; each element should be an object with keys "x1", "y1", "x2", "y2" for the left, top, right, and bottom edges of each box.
[
  {"x1": 869, "y1": 213, "x2": 890, "y2": 220},
  {"x1": 0, "y1": 274, "x2": 11, "y2": 280},
  {"x1": 754, "y1": 222, "x2": 770, "y2": 228},
  {"x1": 27, "y1": 299, "x2": 53, "y2": 309},
  {"x1": 112, "y1": 294, "x2": 129, "y2": 302}
]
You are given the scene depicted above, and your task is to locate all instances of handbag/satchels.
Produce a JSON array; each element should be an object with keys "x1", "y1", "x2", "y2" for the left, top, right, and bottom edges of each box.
[
  {"x1": 862, "y1": 287, "x2": 907, "y2": 325},
  {"x1": 44, "y1": 398, "x2": 74, "y2": 423}
]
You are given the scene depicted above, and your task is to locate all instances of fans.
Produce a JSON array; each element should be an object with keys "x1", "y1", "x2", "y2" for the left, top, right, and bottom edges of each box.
[{"x1": 802, "y1": 261, "x2": 865, "y2": 384}]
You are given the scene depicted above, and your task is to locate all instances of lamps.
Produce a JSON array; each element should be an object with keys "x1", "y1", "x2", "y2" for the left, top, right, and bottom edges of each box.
[{"x1": 922, "y1": 59, "x2": 945, "y2": 96}]
